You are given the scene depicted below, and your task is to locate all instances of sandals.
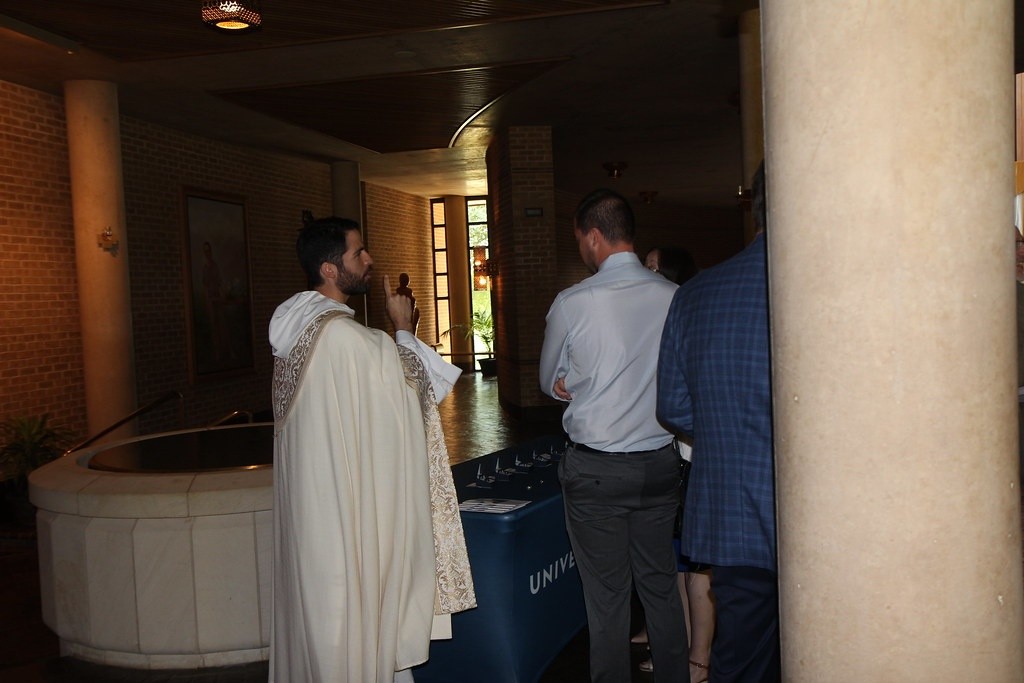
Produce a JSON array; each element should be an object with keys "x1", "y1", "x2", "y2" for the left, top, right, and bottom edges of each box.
[
  {"x1": 689, "y1": 660, "x2": 711, "y2": 683},
  {"x1": 640, "y1": 658, "x2": 653, "y2": 672}
]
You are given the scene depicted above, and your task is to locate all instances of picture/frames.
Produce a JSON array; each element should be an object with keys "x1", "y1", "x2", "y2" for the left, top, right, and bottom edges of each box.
[{"x1": 178, "y1": 185, "x2": 262, "y2": 385}]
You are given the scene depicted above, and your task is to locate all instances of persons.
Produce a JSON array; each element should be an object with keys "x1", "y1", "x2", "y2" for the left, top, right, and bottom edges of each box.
[
  {"x1": 538, "y1": 186, "x2": 691, "y2": 683},
  {"x1": 396, "y1": 273, "x2": 420, "y2": 336},
  {"x1": 623, "y1": 157, "x2": 781, "y2": 683},
  {"x1": 265, "y1": 216, "x2": 478, "y2": 683}
]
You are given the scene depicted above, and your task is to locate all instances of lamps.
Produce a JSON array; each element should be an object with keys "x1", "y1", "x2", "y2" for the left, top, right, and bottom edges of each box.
[
  {"x1": 603, "y1": 160, "x2": 628, "y2": 179},
  {"x1": 736, "y1": 185, "x2": 753, "y2": 212},
  {"x1": 97, "y1": 226, "x2": 118, "y2": 249},
  {"x1": 201, "y1": 0, "x2": 262, "y2": 34},
  {"x1": 640, "y1": 190, "x2": 658, "y2": 205},
  {"x1": 475, "y1": 259, "x2": 499, "y2": 285}
]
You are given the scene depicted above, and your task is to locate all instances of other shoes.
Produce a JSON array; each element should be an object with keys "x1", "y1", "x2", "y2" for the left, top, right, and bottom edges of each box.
[{"x1": 630, "y1": 636, "x2": 651, "y2": 651}]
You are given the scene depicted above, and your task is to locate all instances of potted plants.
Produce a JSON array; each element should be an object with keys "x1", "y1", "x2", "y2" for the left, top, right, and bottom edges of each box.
[
  {"x1": 0, "y1": 413, "x2": 81, "y2": 527},
  {"x1": 441, "y1": 313, "x2": 497, "y2": 377}
]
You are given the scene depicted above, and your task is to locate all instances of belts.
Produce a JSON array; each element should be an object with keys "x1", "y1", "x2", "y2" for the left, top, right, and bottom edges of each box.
[{"x1": 567, "y1": 434, "x2": 672, "y2": 456}]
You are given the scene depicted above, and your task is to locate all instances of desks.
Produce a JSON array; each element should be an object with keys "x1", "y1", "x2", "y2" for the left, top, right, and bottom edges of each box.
[{"x1": 410, "y1": 434, "x2": 587, "y2": 683}]
